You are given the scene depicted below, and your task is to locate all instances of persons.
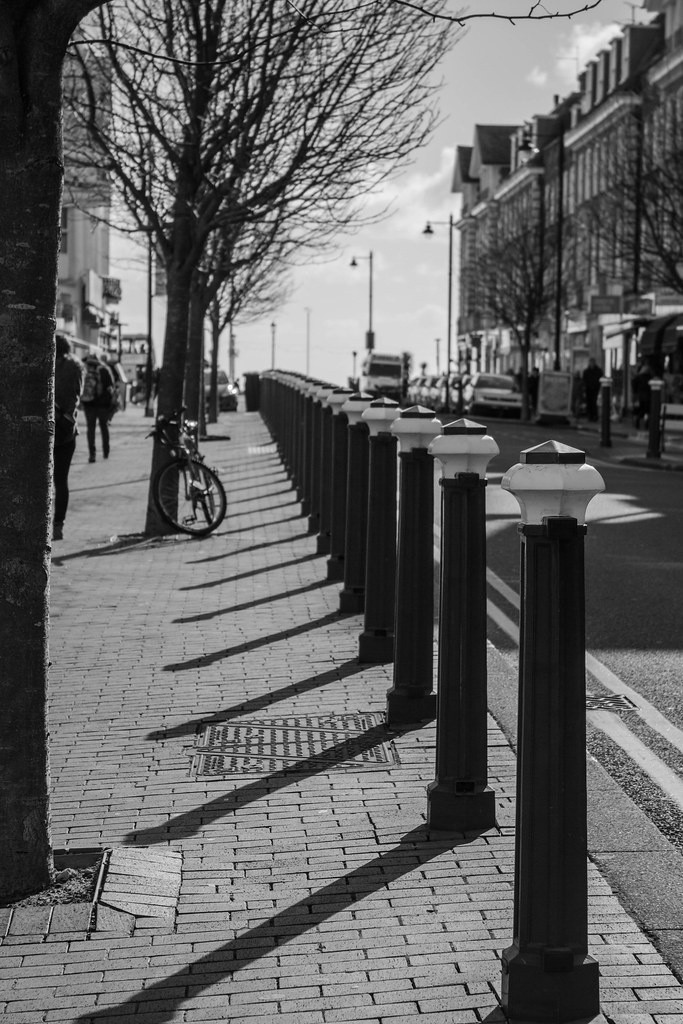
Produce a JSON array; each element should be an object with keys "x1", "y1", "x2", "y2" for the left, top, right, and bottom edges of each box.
[
  {"x1": 48, "y1": 331, "x2": 163, "y2": 544},
  {"x1": 631, "y1": 358, "x2": 654, "y2": 430},
  {"x1": 504, "y1": 356, "x2": 604, "y2": 424}
]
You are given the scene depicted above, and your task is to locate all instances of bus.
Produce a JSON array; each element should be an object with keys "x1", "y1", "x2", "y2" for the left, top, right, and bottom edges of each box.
[{"x1": 363, "y1": 353, "x2": 404, "y2": 402}]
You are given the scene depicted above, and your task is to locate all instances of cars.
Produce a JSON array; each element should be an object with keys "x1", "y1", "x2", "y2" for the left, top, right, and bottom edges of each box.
[
  {"x1": 463, "y1": 373, "x2": 522, "y2": 415},
  {"x1": 201, "y1": 367, "x2": 238, "y2": 410},
  {"x1": 407, "y1": 371, "x2": 471, "y2": 409}
]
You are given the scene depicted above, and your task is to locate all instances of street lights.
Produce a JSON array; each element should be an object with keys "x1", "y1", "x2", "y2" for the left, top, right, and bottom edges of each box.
[
  {"x1": 419, "y1": 213, "x2": 453, "y2": 411},
  {"x1": 349, "y1": 250, "x2": 374, "y2": 354}
]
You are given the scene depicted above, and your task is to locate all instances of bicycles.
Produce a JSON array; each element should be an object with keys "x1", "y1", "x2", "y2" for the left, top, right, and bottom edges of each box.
[{"x1": 141, "y1": 404, "x2": 227, "y2": 536}]
